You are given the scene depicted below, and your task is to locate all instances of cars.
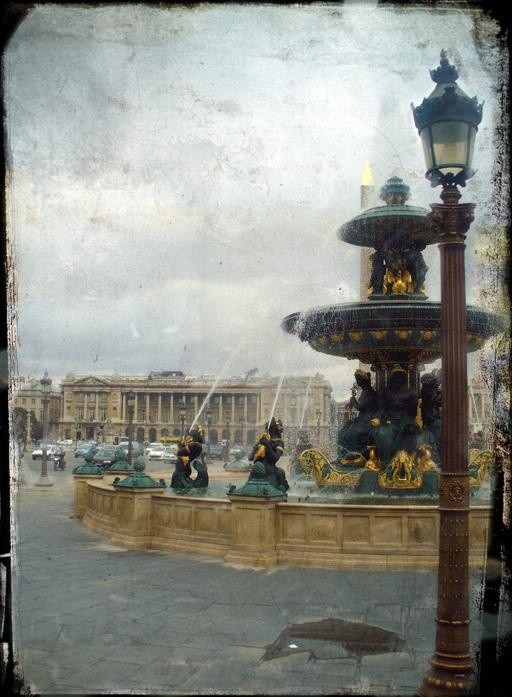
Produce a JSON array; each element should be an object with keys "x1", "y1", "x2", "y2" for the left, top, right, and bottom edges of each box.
[
  {"x1": 31, "y1": 444, "x2": 60, "y2": 460},
  {"x1": 75, "y1": 440, "x2": 145, "y2": 464},
  {"x1": 198, "y1": 444, "x2": 253, "y2": 459},
  {"x1": 146, "y1": 442, "x2": 180, "y2": 463}
]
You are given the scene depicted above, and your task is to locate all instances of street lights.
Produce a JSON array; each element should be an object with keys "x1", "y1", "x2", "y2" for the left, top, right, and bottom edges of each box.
[
  {"x1": 316, "y1": 409, "x2": 322, "y2": 448},
  {"x1": 225, "y1": 415, "x2": 230, "y2": 459},
  {"x1": 180, "y1": 406, "x2": 186, "y2": 440},
  {"x1": 409, "y1": 49, "x2": 485, "y2": 680},
  {"x1": 39, "y1": 370, "x2": 52, "y2": 476},
  {"x1": 126, "y1": 391, "x2": 135, "y2": 464},
  {"x1": 206, "y1": 407, "x2": 211, "y2": 454},
  {"x1": 74, "y1": 415, "x2": 76, "y2": 447},
  {"x1": 239, "y1": 416, "x2": 244, "y2": 448}
]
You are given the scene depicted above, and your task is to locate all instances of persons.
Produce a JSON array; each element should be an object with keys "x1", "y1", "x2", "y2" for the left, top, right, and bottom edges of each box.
[
  {"x1": 335, "y1": 369, "x2": 377, "y2": 463},
  {"x1": 248, "y1": 416, "x2": 290, "y2": 493},
  {"x1": 370, "y1": 364, "x2": 433, "y2": 466},
  {"x1": 54, "y1": 446, "x2": 66, "y2": 467},
  {"x1": 420, "y1": 373, "x2": 443, "y2": 443},
  {"x1": 177, "y1": 427, "x2": 205, "y2": 476},
  {"x1": 370, "y1": 238, "x2": 429, "y2": 295}
]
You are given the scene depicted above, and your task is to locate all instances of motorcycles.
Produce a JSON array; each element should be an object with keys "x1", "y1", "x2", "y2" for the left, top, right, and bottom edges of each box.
[{"x1": 53, "y1": 452, "x2": 66, "y2": 471}]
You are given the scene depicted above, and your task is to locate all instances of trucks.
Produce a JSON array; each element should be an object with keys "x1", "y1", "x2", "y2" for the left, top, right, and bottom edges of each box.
[{"x1": 59, "y1": 440, "x2": 72, "y2": 445}]
